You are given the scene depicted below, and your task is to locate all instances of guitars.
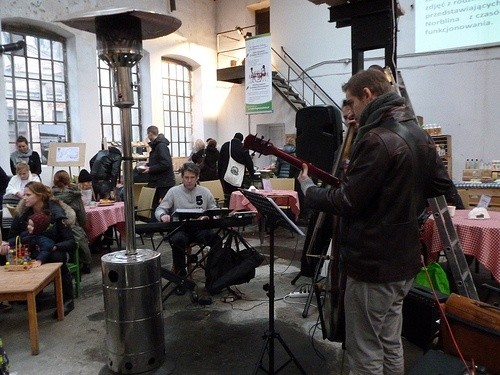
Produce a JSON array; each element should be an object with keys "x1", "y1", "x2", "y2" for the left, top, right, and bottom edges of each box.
[{"x1": 242, "y1": 134, "x2": 429, "y2": 231}]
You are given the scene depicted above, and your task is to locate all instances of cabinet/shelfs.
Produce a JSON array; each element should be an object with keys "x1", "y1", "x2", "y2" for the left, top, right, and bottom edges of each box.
[{"x1": 430, "y1": 135, "x2": 451, "y2": 179}]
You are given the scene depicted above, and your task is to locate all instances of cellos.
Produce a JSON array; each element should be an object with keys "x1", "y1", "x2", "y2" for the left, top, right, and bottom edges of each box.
[{"x1": 311, "y1": 66, "x2": 385, "y2": 375}]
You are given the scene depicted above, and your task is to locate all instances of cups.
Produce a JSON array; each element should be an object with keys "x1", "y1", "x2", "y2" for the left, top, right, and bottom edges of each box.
[
  {"x1": 89, "y1": 201, "x2": 98, "y2": 208},
  {"x1": 447, "y1": 206, "x2": 456, "y2": 219}
]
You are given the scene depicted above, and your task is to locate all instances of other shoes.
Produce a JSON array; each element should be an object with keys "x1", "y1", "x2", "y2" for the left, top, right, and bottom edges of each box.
[
  {"x1": 80, "y1": 262, "x2": 91, "y2": 274},
  {"x1": 175, "y1": 283, "x2": 186, "y2": 295},
  {"x1": 101, "y1": 238, "x2": 114, "y2": 245},
  {"x1": 64, "y1": 297, "x2": 75, "y2": 316}
]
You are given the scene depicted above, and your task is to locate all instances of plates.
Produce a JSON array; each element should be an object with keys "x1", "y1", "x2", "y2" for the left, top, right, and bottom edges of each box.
[{"x1": 98, "y1": 200, "x2": 115, "y2": 206}]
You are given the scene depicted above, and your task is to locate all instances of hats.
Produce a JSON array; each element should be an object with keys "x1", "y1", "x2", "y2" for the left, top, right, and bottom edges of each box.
[
  {"x1": 29, "y1": 212, "x2": 51, "y2": 233},
  {"x1": 78, "y1": 169, "x2": 93, "y2": 183}
]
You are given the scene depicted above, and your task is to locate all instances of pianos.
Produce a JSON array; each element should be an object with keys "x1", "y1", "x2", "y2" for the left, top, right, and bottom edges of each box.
[{"x1": 135, "y1": 215, "x2": 254, "y2": 310}]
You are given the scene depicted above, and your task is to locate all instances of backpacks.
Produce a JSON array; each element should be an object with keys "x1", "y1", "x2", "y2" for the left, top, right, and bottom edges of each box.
[{"x1": 204, "y1": 233, "x2": 266, "y2": 296}]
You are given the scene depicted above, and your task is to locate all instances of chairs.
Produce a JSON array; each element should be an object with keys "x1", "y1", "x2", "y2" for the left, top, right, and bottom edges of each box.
[
  {"x1": 106, "y1": 183, "x2": 150, "y2": 242},
  {"x1": 200, "y1": 179, "x2": 230, "y2": 206},
  {"x1": 117, "y1": 186, "x2": 158, "y2": 250},
  {"x1": 66, "y1": 240, "x2": 80, "y2": 299},
  {"x1": 264, "y1": 177, "x2": 295, "y2": 238}
]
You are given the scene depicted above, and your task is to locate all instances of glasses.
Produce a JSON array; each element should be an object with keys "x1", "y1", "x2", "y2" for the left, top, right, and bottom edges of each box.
[{"x1": 182, "y1": 176, "x2": 196, "y2": 179}]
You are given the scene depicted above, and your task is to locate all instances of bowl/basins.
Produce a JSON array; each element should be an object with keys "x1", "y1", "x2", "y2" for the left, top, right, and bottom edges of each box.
[{"x1": 135, "y1": 166, "x2": 147, "y2": 174}]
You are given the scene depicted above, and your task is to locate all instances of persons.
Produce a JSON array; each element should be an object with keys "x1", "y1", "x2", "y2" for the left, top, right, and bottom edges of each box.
[
  {"x1": 297, "y1": 70, "x2": 474, "y2": 375},
  {"x1": 0, "y1": 125, "x2": 296, "y2": 317}
]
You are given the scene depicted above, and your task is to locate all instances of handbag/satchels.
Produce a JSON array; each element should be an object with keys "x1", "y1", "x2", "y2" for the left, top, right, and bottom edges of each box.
[
  {"x1": 224, "y1": 156, "x2": 245, "y2": 187},
  {"x1": 416, "y1": 261, "x2": 450, "y2": 296},
  {"x1": 192, "y1": 152, "x2": 206, "y2": 168}
]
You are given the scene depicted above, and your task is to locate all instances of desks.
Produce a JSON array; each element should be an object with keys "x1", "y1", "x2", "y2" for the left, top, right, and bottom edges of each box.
[
  {"x1": 0, "y1": 262, "x2": 64, "y2": 355},
  {"x1": 232, "y1": 191, "x2": 300, "y2": 236},
  {"x1": 421, "y1": 208, "x2": 500, "y2": 282},
  {"x1": 83, "y1": 199, "x2": 125, "y2": 254}
]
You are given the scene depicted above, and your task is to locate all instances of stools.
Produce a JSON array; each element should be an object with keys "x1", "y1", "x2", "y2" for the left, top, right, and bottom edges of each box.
[{"x1": 168, "y1": 239, "x2": 206, "y2": 276}]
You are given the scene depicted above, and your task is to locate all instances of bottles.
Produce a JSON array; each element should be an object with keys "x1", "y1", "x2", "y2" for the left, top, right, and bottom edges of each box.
[
  {"x1": 466, "y1": 158, "x2": 480, "y2": 170},
  {"x1": 422, "y1": 123, "x2": 441, "y2": 137},
  {"x1": 436, "y1": 143, "x2": 446, "y2": 157}
]
